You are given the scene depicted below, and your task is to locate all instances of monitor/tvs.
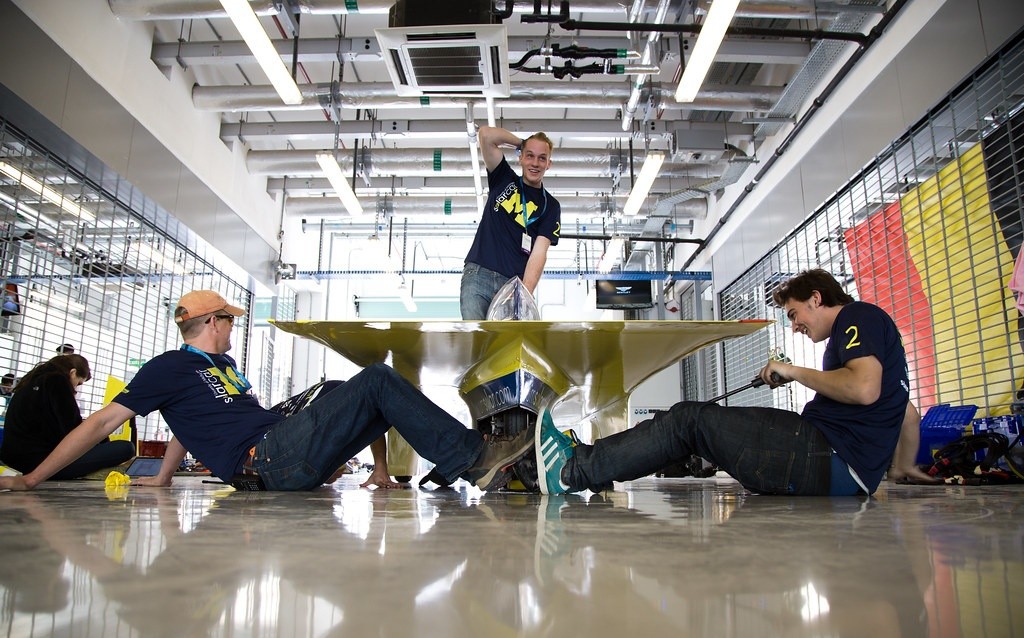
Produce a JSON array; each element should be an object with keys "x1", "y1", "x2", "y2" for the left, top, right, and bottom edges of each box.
[
  {"x1": 0, "y1": 283, "x2": 20, "y2": 316},
  {"x1": 596, "y1": 279, "x2": 653, "y2": 310}
]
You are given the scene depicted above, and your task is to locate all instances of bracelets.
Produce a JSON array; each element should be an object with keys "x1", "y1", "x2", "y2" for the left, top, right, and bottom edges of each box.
[{"x1": 516, "y1": 139, "x2": 524, "y2": 151}]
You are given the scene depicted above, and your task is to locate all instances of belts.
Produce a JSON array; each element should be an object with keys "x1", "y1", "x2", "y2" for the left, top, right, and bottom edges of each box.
[{"x1": 245, "y1": 453, "x2": 253, "y2": 479}]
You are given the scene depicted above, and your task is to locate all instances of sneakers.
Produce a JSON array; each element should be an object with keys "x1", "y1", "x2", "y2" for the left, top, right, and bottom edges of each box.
[
  {"x1": 468, "y1": 422, "x2": 536, "y2": 492},
  {"x1": 535, "y1": 406, "x2": 575, "y2": 495}
]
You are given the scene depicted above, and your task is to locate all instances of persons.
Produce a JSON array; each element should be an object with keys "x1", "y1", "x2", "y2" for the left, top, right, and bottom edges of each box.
[
  {"x1": 0, "y1": 343, "x2": 136, "y2": 481},
  {"x1": 459, "y1": 127, "x2": 561, "y2": 320},
  {"x1": 0, "y1": 372, "x2": 14, "y2": 397},
  {"x1": 0, "y1": 490, "x2": 531, "y2": 638},
  {"x1": 0, "y1": 290, "x2": 534, "y2": 491},
  {"x1": 533, "y1": 494, "x2": 932, "y2": 638},
  {"x1": 534, "y1": 269, "x2": 941, "y2": 498}
]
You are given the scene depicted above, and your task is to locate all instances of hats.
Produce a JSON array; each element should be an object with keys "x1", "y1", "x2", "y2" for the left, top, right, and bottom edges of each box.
[
  {"x1": 175, "y1": 290, "x2": 245, "y2": 323},
  {"x1": 418, "y1": 465, "x2": 457, "y2": 486}
]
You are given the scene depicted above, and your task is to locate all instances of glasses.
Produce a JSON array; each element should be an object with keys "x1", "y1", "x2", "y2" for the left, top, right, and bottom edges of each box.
[{"x1": 205, "y1": 315, "x2": 234, "y2": 327}]
